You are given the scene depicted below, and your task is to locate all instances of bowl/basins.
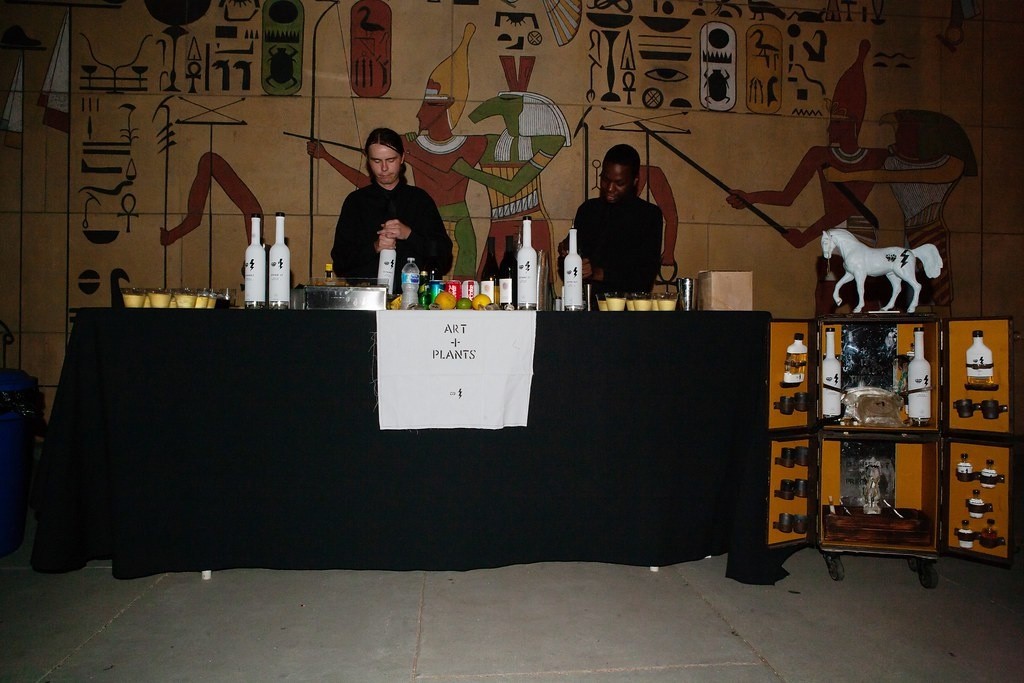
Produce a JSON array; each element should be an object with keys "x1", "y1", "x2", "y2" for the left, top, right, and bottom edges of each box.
[
  {"x1": 596, "y1": 292, "x2": 678, "y2": 311},
  {"x1": 120, "y1": 287, "x2": 230, "y2": 308}
]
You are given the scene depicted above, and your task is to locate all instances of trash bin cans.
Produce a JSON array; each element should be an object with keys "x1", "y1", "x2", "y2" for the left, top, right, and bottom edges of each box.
[{"x1": 0, "y1": 369, "x2": 37, "y2": 556}]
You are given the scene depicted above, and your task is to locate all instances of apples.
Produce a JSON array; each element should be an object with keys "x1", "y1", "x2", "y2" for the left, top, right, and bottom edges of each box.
[
  {"x1": 472, "y1": 294, "x2": 491, "y2": 309},
  {"x1": 435, "y1": 291, "x2": 455, "y2": 309}
]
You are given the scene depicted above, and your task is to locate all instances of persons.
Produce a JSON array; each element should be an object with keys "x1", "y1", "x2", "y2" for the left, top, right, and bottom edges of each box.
[
  {"x1": 330, "y1": 128, "x2": 453, "y2": 297},
  {"x1": 558, "y1": 143, "x2": 664, "y2": 298}
]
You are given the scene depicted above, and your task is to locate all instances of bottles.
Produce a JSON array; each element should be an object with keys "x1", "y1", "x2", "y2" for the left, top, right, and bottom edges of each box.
[
  {"x1": 959, "y1": 520, "x2": 973, "y2": 548},
  {"x1": 969, "y1": 490, "x2": 984, "y2": 518},
  {"x1": 966, "y1": 330, "x2": 994, "y2": 386},
  {"x1": 401, "y1": 257, "x2": 429, "y2": 309},
  {"x1": 980, "y1": 460, "x2": 996, "y2": 488},
  {"x1": 480, "y1": 237, "x2": 500, "y2": 306},
  {"x1": 377, "y1": 249, "x2": 396, "y2": 295},
  {"x1": 500, "y1": 236, "x2": 518, "y2": 309},
  {"x1": 783, "y1": 332, "x2": 807, "y2": 383},
  {"x1": 325, "y1": 264, "x2": 333, "y2": 279},
  {"x1": 245, "y1": 213, "x2": 266, "y2": 309},
  {"x1": 908, "y1": 327, "x2": 930, "y2": 418},
  {"x1": 269, "y1": 212, "x2": 290, "y2": 309},
  {"x1": 583, "y1": 281, "x2": 593, "y2": 311},
  {"x1": 957, "y1": 453, "x2": 972, "y2": 473},
  {"x1": 556, "y1": 297, "x2": 561, "y2": 311},
  {"x1": 822, "y1": 328, "x2": 841, "y2": 415},
  {"x1": 514, "y1": 216, "x2": 553, "y2": 311},
  {"x1": 982, "y1": 519, "x2": 997, "y2": 538},
  {"x1": 564, "y1": 228, "x2": 583, "y2": 311}
]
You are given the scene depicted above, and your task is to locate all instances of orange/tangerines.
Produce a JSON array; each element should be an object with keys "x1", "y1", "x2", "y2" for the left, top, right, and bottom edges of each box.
[{"x1": 457, "y1": 297, "x2": 472, "y2": 309}]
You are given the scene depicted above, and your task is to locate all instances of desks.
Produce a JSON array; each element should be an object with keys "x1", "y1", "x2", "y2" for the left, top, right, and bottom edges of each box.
[{"x1": 33, "y1": 303, "x2": 775, "y2": 597}]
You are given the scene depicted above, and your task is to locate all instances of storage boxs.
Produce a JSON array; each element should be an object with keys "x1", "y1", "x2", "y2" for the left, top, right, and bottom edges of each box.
[
  {"x1": 305, "y1": 284, "x2": 388, "y2": 311},
  {"x1": 289, "y1": 287, "x2": 308, "y2": 310},
  {"x1": 699, "y1": 271, "x2": 754, "y2": 310}
]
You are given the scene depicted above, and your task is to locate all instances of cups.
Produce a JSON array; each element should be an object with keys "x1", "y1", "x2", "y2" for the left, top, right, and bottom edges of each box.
[{"x1": 676, "y1": 278, "x2": 693, "y2": 311}]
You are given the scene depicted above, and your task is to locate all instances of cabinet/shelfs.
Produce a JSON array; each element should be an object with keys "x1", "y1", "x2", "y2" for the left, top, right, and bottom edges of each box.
[{"x1": 761, "y1": 312, "x2": 1024, "y2": 592}]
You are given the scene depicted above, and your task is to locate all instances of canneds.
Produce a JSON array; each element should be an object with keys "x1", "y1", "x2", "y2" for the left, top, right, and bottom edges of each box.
[
  {"x1": 462, "y1": 280, "x2": 479, "y2": 301},
  {"x1": 430, "y1": 280, "x2": 444, "y2": 302},
  {"x1": 446, "y1": 280, "x2": 461, "y2": 301}
]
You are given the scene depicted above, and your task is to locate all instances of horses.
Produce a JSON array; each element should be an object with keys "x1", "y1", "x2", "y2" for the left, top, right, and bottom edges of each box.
[{"x1": 821, "y1": 228, "x2": 943, "y2": 314}]
